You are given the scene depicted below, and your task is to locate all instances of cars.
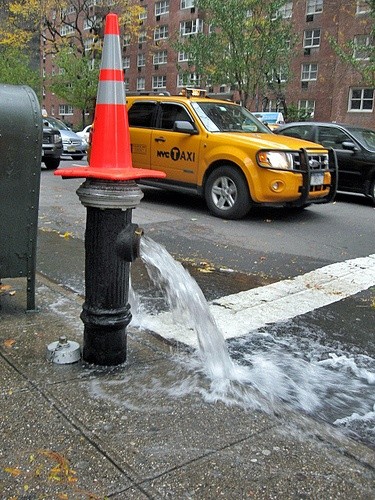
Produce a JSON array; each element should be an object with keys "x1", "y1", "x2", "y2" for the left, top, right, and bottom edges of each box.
[
  {"x1": 271, "y1": 121, "x2": 375, "y2": 203},
  {"x1": 43, "y1": 120, "x2": 89, "y2": 160}
]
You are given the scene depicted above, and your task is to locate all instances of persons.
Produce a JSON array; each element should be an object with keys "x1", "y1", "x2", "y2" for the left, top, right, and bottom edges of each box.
[{"x1": 182, "y1": 88, "x2": 187, "y2": 95}]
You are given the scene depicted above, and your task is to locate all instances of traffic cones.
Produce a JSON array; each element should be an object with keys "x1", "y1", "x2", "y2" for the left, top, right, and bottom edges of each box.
[{"x1": 53, "y1": 11, "x2": 166, "y2": 180}]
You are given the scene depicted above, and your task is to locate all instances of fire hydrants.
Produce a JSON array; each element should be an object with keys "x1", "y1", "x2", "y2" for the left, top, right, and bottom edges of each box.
[{"x1": 74, "y1": 179, "x2": 144, "y2": 370}]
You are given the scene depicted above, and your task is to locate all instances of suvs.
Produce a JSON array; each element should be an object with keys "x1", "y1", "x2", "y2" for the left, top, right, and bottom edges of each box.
[
  {"x1": 87, "y1": 95, "x2": 339, "y2": 221},
  {"x1": 40, "y1": 121, "x2": 62, "y2": 170}
]
